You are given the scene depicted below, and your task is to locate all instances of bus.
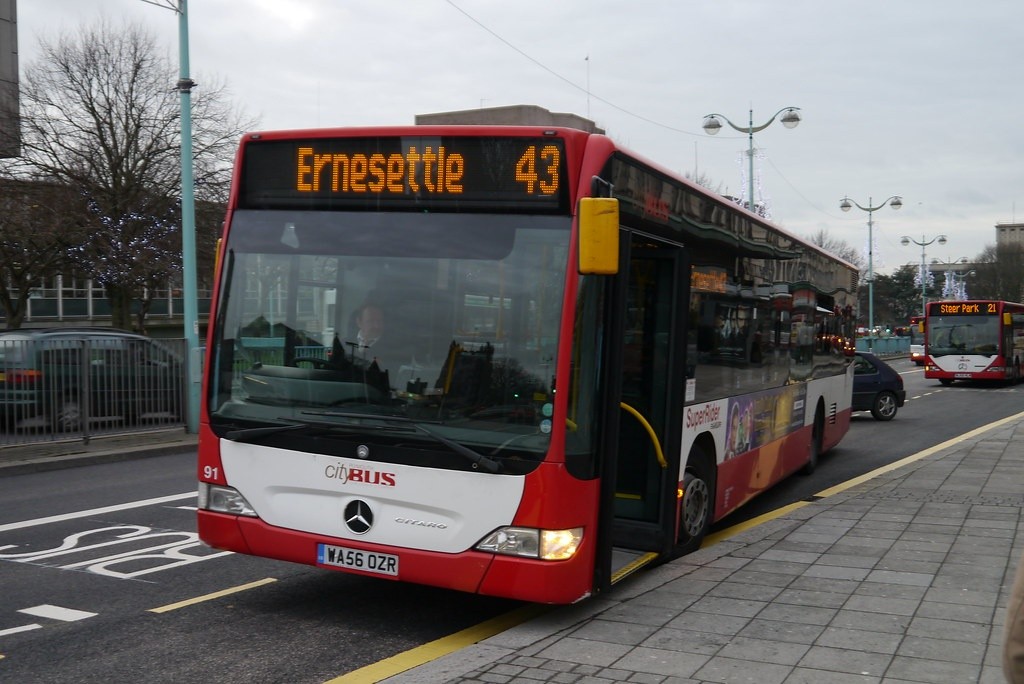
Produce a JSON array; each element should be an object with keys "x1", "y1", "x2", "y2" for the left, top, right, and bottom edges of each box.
[
  {"x1": 195, "y1": 104, "x2": 861, "y2": 607},
  {"x1": 918, "y1": 299, "x2": 1024, "y2": 387},
  {"x1": 909, "y1": 324, "x2": 926, "y2": 366}
]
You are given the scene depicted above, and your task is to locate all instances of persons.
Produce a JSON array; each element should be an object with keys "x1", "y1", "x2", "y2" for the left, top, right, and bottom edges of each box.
[
  {"x1": 340, "y1": 304, "x2": 403, "y2": 372},
  {"x1": 726, "y1": 402, "x2": 739, "y2": 455}
]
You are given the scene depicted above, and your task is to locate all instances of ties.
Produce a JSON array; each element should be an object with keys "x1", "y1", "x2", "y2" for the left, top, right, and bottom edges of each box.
[{"x1": 362, "y1": 340, "x2": 373, "y2": 353}]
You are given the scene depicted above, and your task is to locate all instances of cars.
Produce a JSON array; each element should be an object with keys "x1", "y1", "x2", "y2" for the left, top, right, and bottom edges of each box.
[
  {"x1": 854, "y1": 323, "x2": 910, "y2": 338},
  {"x1": 0, "y1": 326, "x2": 186, "y2": 435},
  {"x1": 851, "y1": 350, "x2": 906, "y2": 422}
]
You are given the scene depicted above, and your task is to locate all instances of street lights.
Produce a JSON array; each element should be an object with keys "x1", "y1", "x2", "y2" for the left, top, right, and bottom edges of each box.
[
  {"x1": 932, "y1": 256, "x2": 968, "y2": 300},
  {"x1": 947, "y1": 269, "x2": 977, "y2": 299},
  {"x1": 700, "y1": 105, "x2": 803, "y2": 213},
  {"x1": 900, "y1": 232, "x2": 949, "y2": 317},
  {"x1": 839, "y1": 195, "x2": 904, "y2": 336}
]
